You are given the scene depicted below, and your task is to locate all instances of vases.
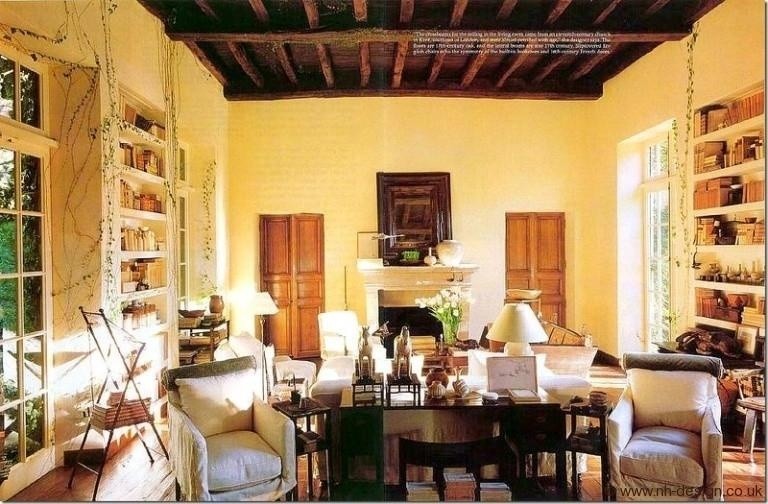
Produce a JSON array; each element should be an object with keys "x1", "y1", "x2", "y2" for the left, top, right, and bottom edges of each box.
[{"x1": 442, "y1": 320, "x2": 460, "y2": 344}]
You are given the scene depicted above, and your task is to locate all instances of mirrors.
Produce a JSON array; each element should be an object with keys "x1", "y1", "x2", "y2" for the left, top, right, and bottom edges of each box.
[{"x1": 376, "y1": 173, "x2": 452, "y2": 264}]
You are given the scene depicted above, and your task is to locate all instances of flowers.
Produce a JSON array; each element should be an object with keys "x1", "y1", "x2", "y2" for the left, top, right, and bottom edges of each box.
[{"x1": 415, "y1": 287, "x2": 474, "y2": 323}]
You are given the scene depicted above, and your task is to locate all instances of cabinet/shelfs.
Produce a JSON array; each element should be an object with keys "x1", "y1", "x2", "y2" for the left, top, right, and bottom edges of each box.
[
  {"x1": 119, "y1": 90, "x2": 168, "y2": 376},
  {"x1": 180, "y1": 320, "x2": 229, "y2": 364},
  {"x1": 692, "y1": 82, "x2": 765, "y2": 361},
  {"x1": 66, "y1": 308, "x2": 170, "y2": 502}
]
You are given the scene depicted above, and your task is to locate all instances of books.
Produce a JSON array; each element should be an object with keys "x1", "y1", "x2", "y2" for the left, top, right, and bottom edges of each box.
[
  {"x1": 507, "y1": 389, "x2": 541, "y2": 402},
  {"x1": 739, "y1": 374, "x2": 764, "y2": 398},
  {"x1": 755, "y1": 336, "x2": 764, "y2": 361},
  {"x1": 121, "y1": 226, "x2": 158, "y2": 251},
  {"x1": 178, "y1": 313, "x2": 228, "y2": 366},
  {"x1": 119, "y1": 140, "x2": 162, "y2": 174},
  {"x1": 694, "y1": 91, "x2": 765, "y2": 246},
  {"x1": 91, "y1": 389, "x2": 152, "y2": 430},
  {"x1": 120, "y1": 180, "x2": 162, "y2": 212},
  {"x1": 696, "y1": 288, "x2": 766, "y2": 328},
  {"x1": 298, "y1": 431, "x2": 321, "y2": 444},
  {"x1": 405, "y1": 472, "x2": 512, "y2": 503}
]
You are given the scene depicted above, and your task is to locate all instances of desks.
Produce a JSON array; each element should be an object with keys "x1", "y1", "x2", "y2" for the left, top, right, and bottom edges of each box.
[
  {"x1": 651, "y1": 340, "x2": 764, "y2": 375},
  {"x1": 736, "y1": 397, "x2": 765, "y2": 453},
  {"x1": 340, "y1": 386, "x2": 566, "y2": 498},
  {"x1": 414, "y1": 343, "x2": 469, "y2": 380}
]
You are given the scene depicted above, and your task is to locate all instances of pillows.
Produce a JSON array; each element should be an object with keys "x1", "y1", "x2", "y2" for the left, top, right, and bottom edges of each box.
[
  {"x1": 228, "y1": 332, "x2": 263, "y2": 362},
  {"x1": 175, "y1": 368, "x2": 256, "y2": 437}
]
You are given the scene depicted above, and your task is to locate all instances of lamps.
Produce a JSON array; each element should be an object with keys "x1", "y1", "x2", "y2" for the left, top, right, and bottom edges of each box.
[
  {"x1": 250, "y1": 292, "x2": 279, "y2": 343},
  {"x1": 486, "y1": 302, "x2": 547, "y2": 356}
]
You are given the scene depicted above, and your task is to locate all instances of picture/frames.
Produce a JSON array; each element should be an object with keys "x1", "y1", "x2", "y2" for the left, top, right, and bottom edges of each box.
[{"x1": 734, "y1": 323, "x2": 759, "y2": 356}]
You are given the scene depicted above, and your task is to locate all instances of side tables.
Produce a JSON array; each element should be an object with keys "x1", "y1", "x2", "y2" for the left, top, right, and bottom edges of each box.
[
  {"x1": 271, "y1": 398, "x2": 333, "y2": 502},
  {"x1": 570, "y1": 395, "x2": 612, "y2": 503}
]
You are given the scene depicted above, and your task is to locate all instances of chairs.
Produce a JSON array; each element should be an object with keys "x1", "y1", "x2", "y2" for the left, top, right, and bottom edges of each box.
[
  {"x1": 214, "y1": 335, "x2": 317, "y2": 392},
  {"x1": 606, "y1": 353, "x2": 724, "y2": 503},
  {"x1": 318, "y1": 310, "x2": 361, "y2": 361},
  {"x1": 398, "y1": 436, "x2": 504, "y2": 502},
  {"x1": 161, "y1": 355, "x2": 298, "y2": 502}
]
